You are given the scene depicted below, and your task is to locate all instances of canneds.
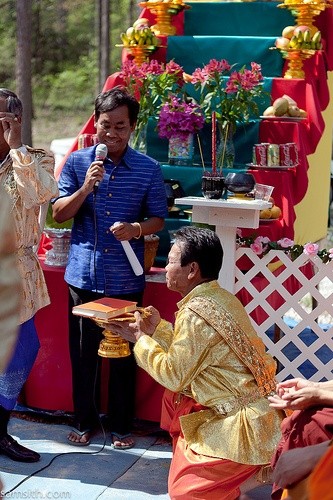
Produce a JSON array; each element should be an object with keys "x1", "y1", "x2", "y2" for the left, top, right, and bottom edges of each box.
[
  {"x1": 78, "y1": 133, "x2": 99, "y2": 151},
  {"x1": 252, "y1": 142, "x2": 299, "y2": 167}
]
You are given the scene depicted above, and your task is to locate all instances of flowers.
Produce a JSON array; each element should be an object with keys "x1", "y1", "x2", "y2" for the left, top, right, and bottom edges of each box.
[
  {"x1": 236, "y1": 227, "x2": 333, "y2": 267},
  {"x1": 118, "y1": 59, "x2": 274, "y2": 139}
]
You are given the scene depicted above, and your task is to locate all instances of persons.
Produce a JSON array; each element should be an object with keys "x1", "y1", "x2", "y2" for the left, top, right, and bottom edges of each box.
[
  {"x1": 0, "y1": 190, "x2": 24, "y2": 500},
  {"x1": 271, "y1": 377, "x2": 333, "y2": 500},
  {"x1": 50, "y1": 85, "x2": 168, "y2": 449},
  {"x1": 0, "y1": 88, "x2": 59, "y2": 463},
  {"x1": 102, "y1": 227, "x2": 287, "y2": 500}
]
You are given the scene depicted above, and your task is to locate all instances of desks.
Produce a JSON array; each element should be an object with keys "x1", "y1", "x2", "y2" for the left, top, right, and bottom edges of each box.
[{"x1": 15, "y1": 250, "x2": 178, "y2": 426}]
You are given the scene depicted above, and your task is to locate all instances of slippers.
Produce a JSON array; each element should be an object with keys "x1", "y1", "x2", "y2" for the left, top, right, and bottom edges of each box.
[
  {"x1": 111, "y1": 432, "x2": 135, "y2": 450},
  {"x1": 66, "y1": 424, "x2": 92, "y2": 447}
]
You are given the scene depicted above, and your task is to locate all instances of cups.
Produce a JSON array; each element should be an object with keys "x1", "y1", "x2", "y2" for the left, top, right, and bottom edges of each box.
[{"x1": 255, "y1": 184, "x2": 274, "y2": 203}]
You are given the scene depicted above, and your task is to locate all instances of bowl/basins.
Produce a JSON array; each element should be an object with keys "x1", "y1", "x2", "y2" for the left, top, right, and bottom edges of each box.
[
  {"x1": 168, "y1": 207, "x2": 180, "y2": 218},
  {"x1": 225, "y1": 173, "x2": 255, "y2": 194}
]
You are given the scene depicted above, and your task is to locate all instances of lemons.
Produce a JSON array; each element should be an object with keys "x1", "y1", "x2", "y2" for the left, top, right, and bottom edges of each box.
[{"x1": 260, "y1": 204, "x2": 281, "y2": 219}]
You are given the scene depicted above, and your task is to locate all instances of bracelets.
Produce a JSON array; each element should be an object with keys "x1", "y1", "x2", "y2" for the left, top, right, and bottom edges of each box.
[{"x1": 134, "y1": 222, "x2": 142, "y2": 239}]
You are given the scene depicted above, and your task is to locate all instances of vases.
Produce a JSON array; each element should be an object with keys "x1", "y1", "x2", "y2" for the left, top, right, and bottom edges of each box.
[
  {"x1": 167, "y1": 134, "x2": 196, "y2": 166},
  {"x1": 216, "y1": 129, "x2": 234, "y2": 169},
  {"x1": 126, "y1": 116, "x2": 147, "y2": 155}
]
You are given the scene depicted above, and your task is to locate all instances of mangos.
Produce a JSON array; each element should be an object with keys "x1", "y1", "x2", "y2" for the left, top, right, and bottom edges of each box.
[{"x1": 262, "y1": 97, "x2": 307, "y2": 117}]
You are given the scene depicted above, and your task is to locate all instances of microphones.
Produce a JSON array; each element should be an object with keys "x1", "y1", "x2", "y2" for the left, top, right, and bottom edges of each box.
[{"x1": 92, "y1": 143, "x2": 108, "y2": 195}]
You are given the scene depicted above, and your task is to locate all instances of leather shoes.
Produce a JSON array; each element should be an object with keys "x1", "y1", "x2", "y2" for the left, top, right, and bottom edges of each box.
[{"x1": 0, "y1": 433, "x2": 41, "y2": 463}]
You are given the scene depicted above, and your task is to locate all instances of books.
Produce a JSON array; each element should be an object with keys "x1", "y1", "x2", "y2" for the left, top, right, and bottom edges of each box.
[{"x1": 72, "y1": 297, "x2": 137, "y2": 320}]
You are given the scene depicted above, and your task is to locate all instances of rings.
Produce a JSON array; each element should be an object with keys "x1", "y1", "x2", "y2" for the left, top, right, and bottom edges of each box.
[{"x1": 15, "y1": 118, "x2": 17, "y2": 121}]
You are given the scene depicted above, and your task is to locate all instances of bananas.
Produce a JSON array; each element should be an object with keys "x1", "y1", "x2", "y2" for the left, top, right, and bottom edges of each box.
[
  {"x1": 289, "y1": 30, "x2": 323, "y2": 50},
  {"x1": 120, "y1": 28, "x2": 158, "y2": 46}
]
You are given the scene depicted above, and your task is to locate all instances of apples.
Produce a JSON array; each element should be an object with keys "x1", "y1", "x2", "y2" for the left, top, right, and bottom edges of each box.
[
  {"x1": 125, "y1": 18, "x2": 150, "y2": 36},
  {"x1": 275, "y1": 25, "x2": 312, "y2": 48}
]
